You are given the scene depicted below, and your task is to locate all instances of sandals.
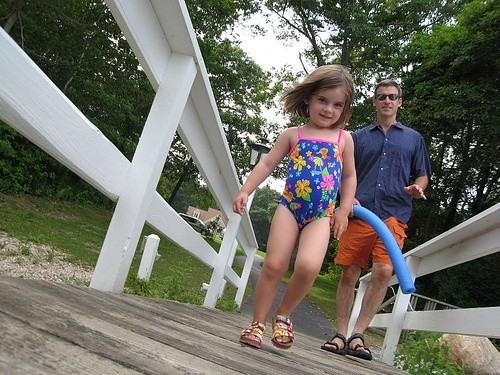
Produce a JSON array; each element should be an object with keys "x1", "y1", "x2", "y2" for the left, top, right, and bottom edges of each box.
[
  {"x1": 346, "y1": 333, "x2": 372, "y2": 360},
  {"x1": 271, "y1": 314, "x2": 295, "y2": 350},
  {"x1": 321, "y1": 333, "x2": 347, "y2": 354},
  {"x1": 240, "y1": 320, "x2": 266, "y2": 349}
]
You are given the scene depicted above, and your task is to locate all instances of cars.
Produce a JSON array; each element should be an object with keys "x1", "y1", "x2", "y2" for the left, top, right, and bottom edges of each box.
[{"x1": 179, "y1": 213, "x2": 210, "y2": 236}]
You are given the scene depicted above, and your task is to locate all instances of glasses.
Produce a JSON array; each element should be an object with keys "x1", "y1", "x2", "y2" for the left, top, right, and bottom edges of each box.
[{"x1": 375, "y1": 94, "x2": 400, "y2": 101}]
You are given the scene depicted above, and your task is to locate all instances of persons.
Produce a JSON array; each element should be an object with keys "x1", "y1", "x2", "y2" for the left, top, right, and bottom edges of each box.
[
  {"x1": 233, "y1": 63, "x2": 359, "y2": 350},
  {"x1": 320, "y1": 78, "x2": 431, "y2": 361}
]
